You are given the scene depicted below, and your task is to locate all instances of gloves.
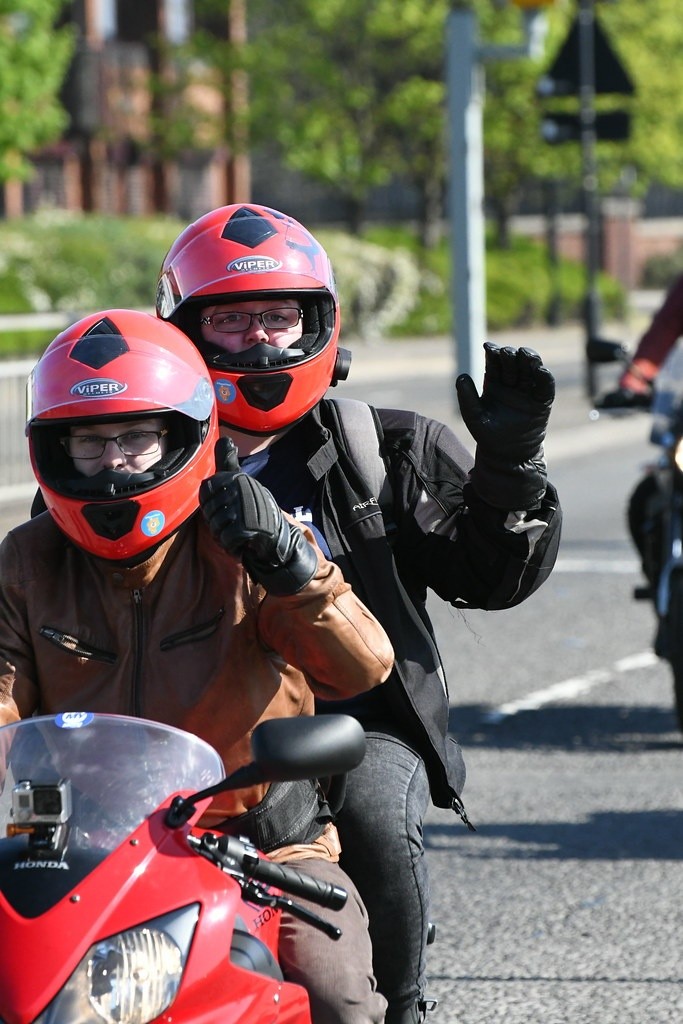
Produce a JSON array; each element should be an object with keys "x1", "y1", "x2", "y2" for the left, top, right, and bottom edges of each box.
[
  {"x1": 456, "y1": 342, "x2": 555, "y2": 508},
  {"x1": 200, "y1": 438, "x2": 316, "y2": 596},
  {"x1": 604, "y1": 386, "x2": 641, "y2": 414}
]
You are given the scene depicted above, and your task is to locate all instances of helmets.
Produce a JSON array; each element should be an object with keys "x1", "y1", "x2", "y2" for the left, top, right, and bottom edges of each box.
[
  {"x1": 27, "y1": 308, "x2": 219, "y2": 561},
  {"x1": 157, "y1": 203, "x2": 342, "y2": 438}
]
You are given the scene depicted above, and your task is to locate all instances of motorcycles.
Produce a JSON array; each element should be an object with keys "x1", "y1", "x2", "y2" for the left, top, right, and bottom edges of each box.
[
  {"x1": 585, "y1": 335, "x2": 683, "y2": 729},
  {"x1": 0, "y1": 715, "x2": 368, "y2": 1024}
]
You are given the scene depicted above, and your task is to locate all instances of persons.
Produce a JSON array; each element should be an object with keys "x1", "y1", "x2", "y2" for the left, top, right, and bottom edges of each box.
[
  {"x1": 153, "y1": 203, "x2": 562, "y2": 1024},
  {"x1": 621, "y1": 270, "x2": 683, "y2": 588},
  {"x1": 0, "y1": 308, "x2": 394, "y2": 1024}
]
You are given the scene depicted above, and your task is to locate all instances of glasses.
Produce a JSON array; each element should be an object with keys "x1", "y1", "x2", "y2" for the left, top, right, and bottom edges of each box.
[
  {"x1": 200, "y1": 307, "x2": 303, "y2": 333},
  {"x1": 59, "y1": 427, "x2": 168, "y2": 460}
]
